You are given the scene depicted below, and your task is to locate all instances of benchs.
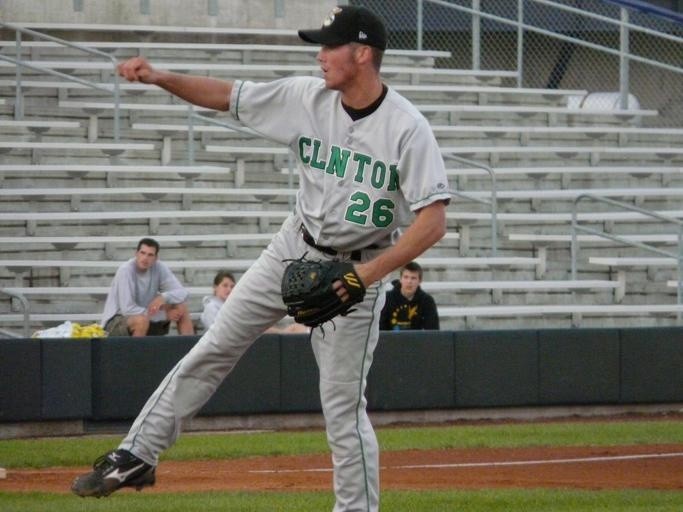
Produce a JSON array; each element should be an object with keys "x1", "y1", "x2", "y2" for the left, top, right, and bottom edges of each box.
[{"x1": 0, "y1": 35, "x2": 683, "y2": 329}]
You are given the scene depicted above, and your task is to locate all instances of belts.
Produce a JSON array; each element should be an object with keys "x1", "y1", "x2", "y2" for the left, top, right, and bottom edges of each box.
[{"x1": 301, "y1": 221, "x2": 363, "y2": 262}]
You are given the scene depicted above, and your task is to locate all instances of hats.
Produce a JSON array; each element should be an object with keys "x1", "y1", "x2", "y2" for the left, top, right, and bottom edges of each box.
[{"x1": 297, "y1": 5, "x2": 388, "y2": 52}]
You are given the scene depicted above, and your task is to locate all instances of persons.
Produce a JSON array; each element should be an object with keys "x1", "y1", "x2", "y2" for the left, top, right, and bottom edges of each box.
[
  {"x1": 378, "y1": 262, "x2": 439, "y2": 332},
  {"x1": 202, "y1": 271, "x2": 308, "y2": 335},
  {"x1": 70, "y1": 4, "x2": 450, "y2": 509},
  {"x1": 101, "y1": 237, "x2": 195, "y2": 337}
]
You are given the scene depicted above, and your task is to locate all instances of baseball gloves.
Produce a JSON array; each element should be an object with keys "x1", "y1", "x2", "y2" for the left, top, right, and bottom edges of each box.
[{"x1": 281, "y1": 250, "x2": 366, "y2": 340}]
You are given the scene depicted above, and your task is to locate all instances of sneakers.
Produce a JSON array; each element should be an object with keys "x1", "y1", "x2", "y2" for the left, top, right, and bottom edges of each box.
[{"x1": 71, "y1": 447, "x2": 158, "y2": 499}]
